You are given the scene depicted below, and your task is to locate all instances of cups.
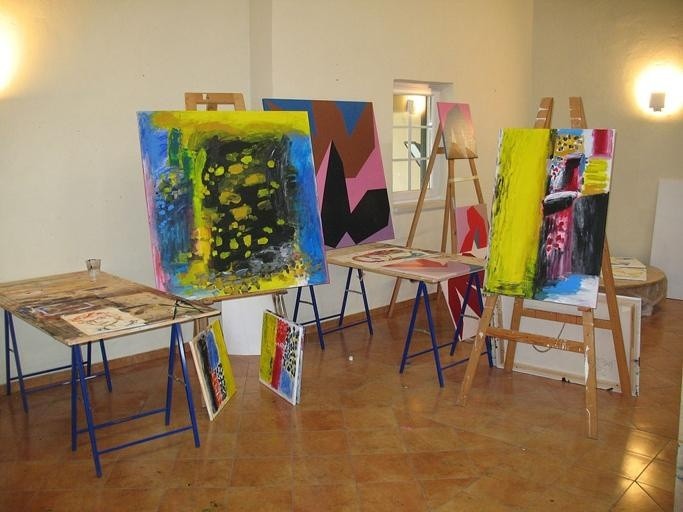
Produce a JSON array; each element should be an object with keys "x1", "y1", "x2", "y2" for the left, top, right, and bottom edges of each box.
[{"x1": 86, "y1": 258, "x2": 101, "y2": 283}]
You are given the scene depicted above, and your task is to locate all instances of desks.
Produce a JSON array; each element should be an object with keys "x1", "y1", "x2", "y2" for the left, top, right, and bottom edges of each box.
[
  {"x1": 1, "y1": 271, "x2": 222, "y2": 477},
  {"x1": 292, "y1": 242, "x2": 493, "y2": 387},
  {"x1": 601, "y1": 261, "x2": 667, "y2": 313}
]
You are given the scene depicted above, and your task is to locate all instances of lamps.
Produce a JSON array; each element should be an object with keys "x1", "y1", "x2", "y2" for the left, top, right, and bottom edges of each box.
[{"x1": 649, "y1": 91, "x2": 666, "y2": 112}]
